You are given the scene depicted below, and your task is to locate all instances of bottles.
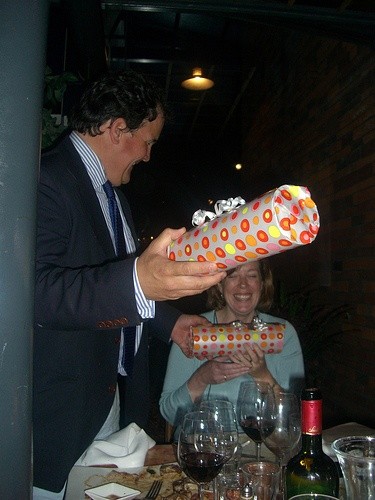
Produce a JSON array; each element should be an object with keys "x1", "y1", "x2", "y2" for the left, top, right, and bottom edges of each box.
[
  {"x1": 240, "y1": 483, "x2": 254, "y2": 500},
  {"x1": 283, "y1": 387, "x2": 339, "y2": 500}
]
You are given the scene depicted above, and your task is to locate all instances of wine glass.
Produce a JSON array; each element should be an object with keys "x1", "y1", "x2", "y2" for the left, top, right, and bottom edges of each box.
[
  {"x1": 257, "y1": 387, "x2": 302, "y2": 499},
  {"x1": 195, "y1": 400, "x2": 238, "y2": 500},
  {"x1": 177, "y1": 410, "x2": 226, "y2": 500},
  {"x1": 235, "y1": 380, "x2": 277, "y2": 461}
]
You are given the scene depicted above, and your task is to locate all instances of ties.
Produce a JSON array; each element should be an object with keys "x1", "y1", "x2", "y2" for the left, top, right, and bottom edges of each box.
[{"x1": 102, "y1": 181, "x2": 136, "y2": 378}]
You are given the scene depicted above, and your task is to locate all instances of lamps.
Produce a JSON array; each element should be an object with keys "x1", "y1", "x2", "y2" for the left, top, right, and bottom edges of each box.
[{"x1": 180, "y1": 69, "x2": 214, "y2": 90}]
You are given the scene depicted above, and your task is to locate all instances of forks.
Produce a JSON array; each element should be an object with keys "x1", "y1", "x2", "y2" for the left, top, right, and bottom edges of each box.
[{"x1": 143, "y1": 480, "x2": 163, "y2": 500}]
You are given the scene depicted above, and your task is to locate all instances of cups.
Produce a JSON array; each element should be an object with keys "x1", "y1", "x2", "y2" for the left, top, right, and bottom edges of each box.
[
  {"x1": 288, "y1": 494, "x2": 339, "y2": 500},
  {"x1": 213, "y1": 464, "x2": 244, "y2": 500},
  {"x1": 331, "y1": 436, "x2": 375, "y2": 500},
  {"x1": 243, "y1": 462, "x2": 283, "y2": 500}
]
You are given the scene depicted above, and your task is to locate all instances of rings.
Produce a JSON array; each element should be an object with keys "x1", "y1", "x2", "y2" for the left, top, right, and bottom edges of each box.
[
  {"x1": 249, "y1": 365, "x2": 253, "y2": 367},
  {"x1": 224, "y1": 376, "x2": 227, "y2": 380}
]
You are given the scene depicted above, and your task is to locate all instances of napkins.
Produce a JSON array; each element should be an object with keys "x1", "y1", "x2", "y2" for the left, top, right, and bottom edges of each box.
[{"x1": 81, "y1": 423, "x2": 156, "y2": 468}]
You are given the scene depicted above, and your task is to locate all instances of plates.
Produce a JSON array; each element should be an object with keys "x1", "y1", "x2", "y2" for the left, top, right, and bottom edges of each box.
[{"x1": 84, "y1": 482, "x2": 141, "y2": 500}]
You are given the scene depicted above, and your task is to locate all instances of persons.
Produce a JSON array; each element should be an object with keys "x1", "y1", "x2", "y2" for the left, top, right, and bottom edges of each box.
[
  {"x1": 32, "y1": 74, "x2": 226, "y2": 500},
  {"x1": 158, "y1": 259, "x2": 304, "y2": 441}
]
